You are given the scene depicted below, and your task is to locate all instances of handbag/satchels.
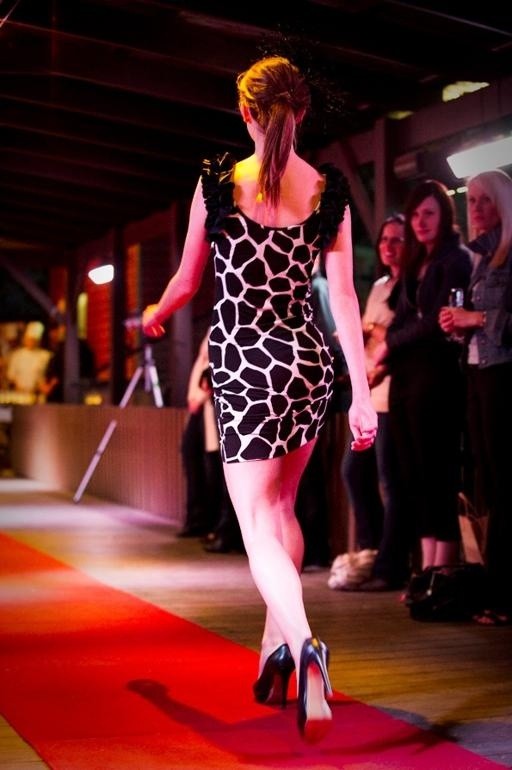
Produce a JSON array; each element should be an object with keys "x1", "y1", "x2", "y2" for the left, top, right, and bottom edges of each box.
[
  {"x1": 406, "y1": 562, "x2": 490, "y2": 623},
  {"x1": 328, "y1": 549, "x2": 378, "y2": 589}
]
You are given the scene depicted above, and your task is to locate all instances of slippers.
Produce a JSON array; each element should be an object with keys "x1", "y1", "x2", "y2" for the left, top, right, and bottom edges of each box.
[{"x1": 473, "y1": 610, "x2": 509, "y2": 626}]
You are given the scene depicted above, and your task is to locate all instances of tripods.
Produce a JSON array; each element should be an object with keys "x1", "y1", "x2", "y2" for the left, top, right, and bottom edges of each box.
[{"x1": 72, "y1": 344, "x2": 164, "y2": 503}]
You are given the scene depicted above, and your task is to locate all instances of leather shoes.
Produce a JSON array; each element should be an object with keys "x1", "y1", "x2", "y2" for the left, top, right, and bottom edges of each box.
[
  {"x1": 345, "y1": 579, "x2": 394, "y2": 591},
  {"x1": 177, "y1": 526, "x2": 236, "y2": 553}
]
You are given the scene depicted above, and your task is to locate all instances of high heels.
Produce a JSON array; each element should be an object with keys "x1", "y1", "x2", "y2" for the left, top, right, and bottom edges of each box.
[
  {"x1": 253, "y1": 644, "x2": 296, "y2": 708},
  {"x1": 296, "y1": 637, "x2": 333, "y2": 743}
]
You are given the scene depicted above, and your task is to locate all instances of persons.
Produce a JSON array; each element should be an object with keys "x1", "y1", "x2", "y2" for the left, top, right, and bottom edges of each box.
[
  {"x1": 364, "y1": 181, "x2": 475, "y2": 620},
  {"x1": 439, "y1": 171, "x2": 508, "y2": 624},
  {"x1": 178, "y1": 318, "x2": 242, "y2": 557},
  {"x1": 1, "y1": 320, "x2": 28, "y2": 478},
  {"x1": 324, "y1": 212, "x2": 406, "y2": 592},
  {"x1": 8, "y1": 322, "x2": 53, "y2": 433},
  {"x1": 140, "y1": 60, "x2": 377, "y2": 743},
  {"x1": 39, "y1": 322, "x2": 94, "y2": 405}
]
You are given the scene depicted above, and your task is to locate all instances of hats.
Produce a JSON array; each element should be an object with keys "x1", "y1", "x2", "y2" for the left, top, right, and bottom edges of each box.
[
  {"x1": 5, "y1": 323, "x2": 18, "y2": 339},
  {"x1": 27, "y1": 321, "x2": 44, "y2": 338}
]
